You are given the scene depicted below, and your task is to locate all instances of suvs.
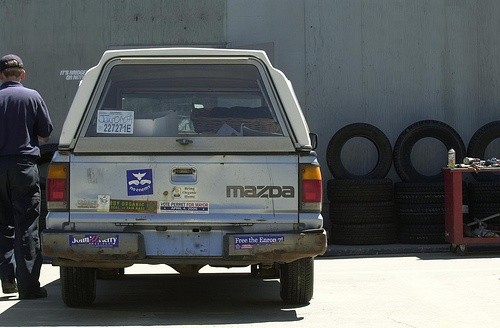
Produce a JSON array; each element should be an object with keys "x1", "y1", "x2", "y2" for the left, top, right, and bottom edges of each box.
[{"x1": 38, "y1": 46, "x2": 328, "y2": 307}]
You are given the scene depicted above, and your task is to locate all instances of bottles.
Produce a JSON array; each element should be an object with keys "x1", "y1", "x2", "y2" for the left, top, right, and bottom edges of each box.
[{"x1": 448, "y1": 147, "x2": 456, "y2": 166}]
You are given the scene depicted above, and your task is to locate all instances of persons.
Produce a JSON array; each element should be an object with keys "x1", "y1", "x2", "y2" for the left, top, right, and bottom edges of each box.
[{"x1": 0, "y1": 54, "x2": 54, "y2": 299}]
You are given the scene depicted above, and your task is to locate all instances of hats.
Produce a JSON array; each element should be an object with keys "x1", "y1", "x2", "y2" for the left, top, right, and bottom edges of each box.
[{"x1": 0, "y1": 54, "x2": 23, "y2": 71}]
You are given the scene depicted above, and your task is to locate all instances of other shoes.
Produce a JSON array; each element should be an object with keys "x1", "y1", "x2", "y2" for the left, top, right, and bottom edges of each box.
[
  {"x1": 2, "y1": 279, "x2": 17, "y2": 293},
  {"x1": 19, "y1": 288, "x2": 48, "y2": 299}
]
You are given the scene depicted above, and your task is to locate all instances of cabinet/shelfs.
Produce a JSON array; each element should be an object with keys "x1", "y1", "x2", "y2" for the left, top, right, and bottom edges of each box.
[{"x1": 440, "y1": 164, "x2": 500, "y2": 255}]
